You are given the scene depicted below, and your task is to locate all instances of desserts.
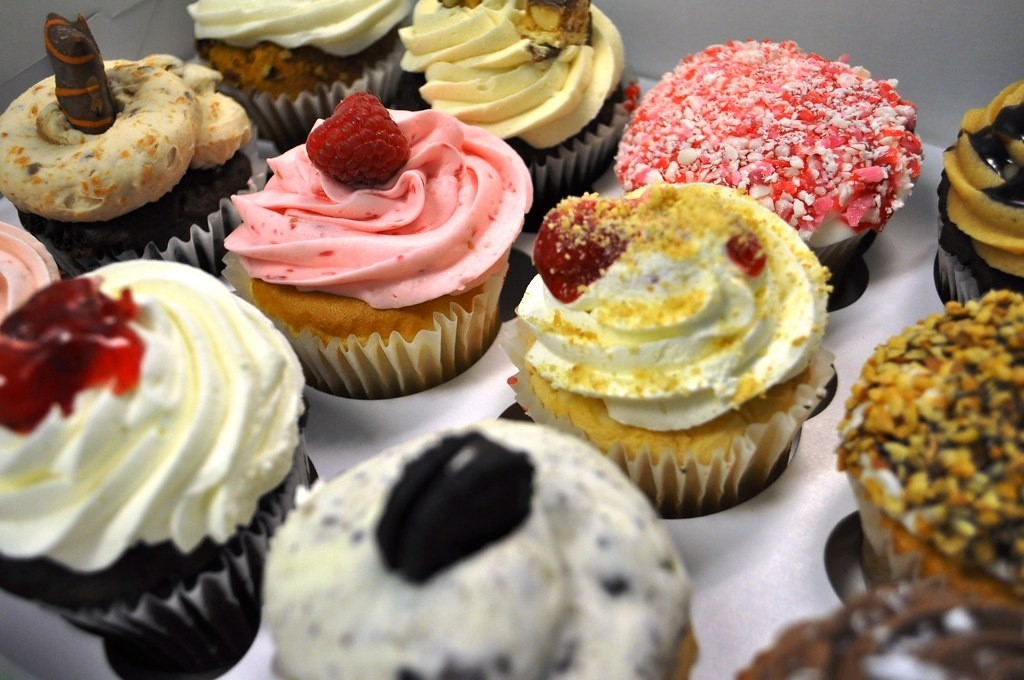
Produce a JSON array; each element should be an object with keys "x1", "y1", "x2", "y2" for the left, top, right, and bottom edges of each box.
[{"x1": 0, "y1": 0, "x2": 1024, "y2": 680}]
